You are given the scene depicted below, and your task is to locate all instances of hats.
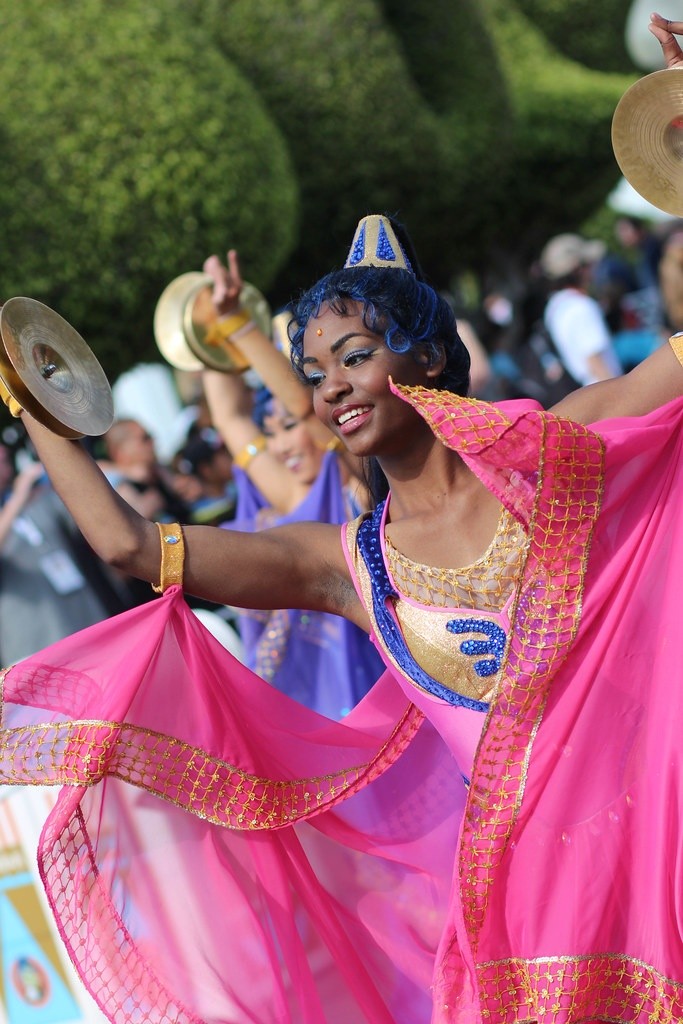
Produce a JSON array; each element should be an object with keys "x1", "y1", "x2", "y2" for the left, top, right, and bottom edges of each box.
[{"x1": 543, "y1": 235, "x2": 608, "y2": 280}]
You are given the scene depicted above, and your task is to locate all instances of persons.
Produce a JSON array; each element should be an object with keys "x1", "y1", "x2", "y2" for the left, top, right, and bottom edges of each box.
[
  {"x1": 0, "y1": 264, "x2": 682, "y2": 1023},
  {"x1": 1, "y1": 213, "x2": 683, "y2": 688}
]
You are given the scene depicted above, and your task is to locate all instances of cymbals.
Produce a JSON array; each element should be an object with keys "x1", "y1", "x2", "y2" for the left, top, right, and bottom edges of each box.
[
  {"x1": 0, "y1": 294, "x2": 114, "y2": 441},
  {"x1": 611, "y1": 67, "x2": 683, "y2": 217},
  {"x1": 152, "y1": 269, "x2": 272, "y2": 373}
]
